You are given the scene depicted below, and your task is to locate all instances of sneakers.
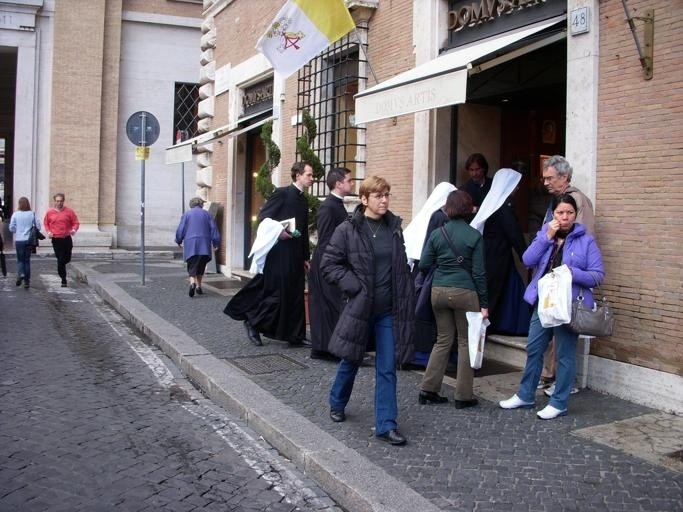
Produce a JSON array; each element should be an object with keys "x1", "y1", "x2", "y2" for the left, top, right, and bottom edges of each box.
[
  {"x1": 545, "y1": 381, "x2": 581, "y2": 397},
  {"x1": 363, "y1": 354, "x2": 374, "y2": 363},
  {"x1": 62, "y1": 280, "x2": 67, "y2": 287},
  {"x1": 330, "y1": 404, "x2": 345, "y2": 422},
  {"x1": 196, "y1": 287, "x2": 202, "y2": 294},
  {"x1": 498, "y1": 392, "x2": 536, "y2": 409},
  {"x1": 16, "y1": 279, "x2": 21, "y2": 286},
  {"x1": 375, "y1": 428, "x2": 407, "y2": 444},
  {"x1": 311, "y1": 348, "x2": 342, "y2": 360},
  {"x1": 24, "y1": 282, "x2": 29, "y2": 288},
  {"x1": 189, "y1": 283, "x2": 196, "y2": 297},
  {"x1": 536, "y1": 377, "x2": 556, "y2": 388},
  {"x1": 536, "y1": 403, "x2": 568, "y2": 420}
]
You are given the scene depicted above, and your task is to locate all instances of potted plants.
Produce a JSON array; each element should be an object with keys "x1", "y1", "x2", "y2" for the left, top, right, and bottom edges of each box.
[{"x1": 296, "y1": 105, "x2": 325, "y2": 325}]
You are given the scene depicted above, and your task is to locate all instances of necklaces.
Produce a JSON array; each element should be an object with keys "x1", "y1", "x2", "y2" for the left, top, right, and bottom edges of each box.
[{"x1": 366, "y1": 217, "x2": 383, "y2": 238}]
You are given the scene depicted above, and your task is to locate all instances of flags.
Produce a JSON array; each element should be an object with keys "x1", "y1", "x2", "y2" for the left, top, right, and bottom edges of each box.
[{"x1": 254, "y1": 1, "x2": 357, "y2": 83}]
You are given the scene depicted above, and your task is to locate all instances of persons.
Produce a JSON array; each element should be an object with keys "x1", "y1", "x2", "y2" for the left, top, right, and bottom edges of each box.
[
  {"x1": 174, "y1": 197, "x2": 220, "y2": 296},
  {"x1": 7, "y1": 197, "x2": 40, "y2": 288},
  {"x1": 496, "y1": 194, "x2": 605, "y2": 421},
  {"x1": 454, "y1": 153, "x2": 493, "y2": 225},
  {"x1": 399, "y1": 180, "x2": 460, "y2": 372},
  {"x1": 43, "y1": 192, "x2": 79, "y2": 287},
  {"x1": 222, "y1": 161, "x2": 314, "y2": 347},
  {"x1": 415, "y1": 189, "x2": 491, "y2": 410},
  {"x1": 467, "y1": 166, "x2": 535, "y2": 336},
  {"x1": 534, "y1": 155, "x2": 596, "y2": 398},
  {"x1": 317, "y1": 174, "x2": 420, "y2": 447},
  {"x1": 304, "y1": 167, "x2": 349, "y2": 364}
]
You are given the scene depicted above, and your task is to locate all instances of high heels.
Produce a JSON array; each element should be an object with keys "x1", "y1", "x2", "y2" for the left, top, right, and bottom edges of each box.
[
  {"x1": 455, "y1": 398, "x2": 478, "y2": 409},
  {"x1": 419, "y1": 390, "x2": 449, "y2": 405}
]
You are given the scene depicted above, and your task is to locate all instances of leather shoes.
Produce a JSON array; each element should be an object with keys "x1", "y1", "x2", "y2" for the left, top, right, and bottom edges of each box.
[
  {"x1": 245, "y1": 320, "x2": 262, "y2": 346},
  {"x1": 288, "y1": 338, "x2": 314, "y2": 348}
]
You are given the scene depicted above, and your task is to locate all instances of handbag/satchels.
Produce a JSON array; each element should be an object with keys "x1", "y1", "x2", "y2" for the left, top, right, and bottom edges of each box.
[
  {"x1": 0, "y1": 252, "x2": 8, "y2": 278},
  {"x1": 32, "y1": 227, "x2": 38, "y2": 246},
  {"x1": 567, "y1": 270, "x2": 615, "y2": 336}
]
